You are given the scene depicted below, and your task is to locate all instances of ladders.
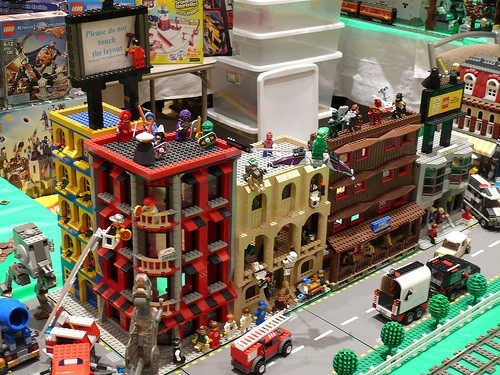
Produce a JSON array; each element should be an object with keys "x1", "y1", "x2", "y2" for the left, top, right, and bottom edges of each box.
[{"x1": 233, "y1": 311, "x2": 293, "y2": 352}]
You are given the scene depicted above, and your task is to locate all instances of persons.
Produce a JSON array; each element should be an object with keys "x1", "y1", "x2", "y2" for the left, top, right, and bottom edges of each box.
[
  {"x1": 328, "y1": 111, "x2": 341, "y2": 139},
  {"x1": 261, "y1": 132, "x2": 277, "y2": 157},
  {"x1": 429, "y1": 223, "x2": 439, "y2": 244},
  {"x1": 449, "y1": 62, "x2": 461, "y2": 84},
  {"x1": 246, "y1": 254, "x2": 271, "y2": 289},
  {"x1": 104, "y1": 213, "x2": 124, "y2": 244},
  {"x1": 296, "y1": 276, "x2": 312, "y2": 302},
  {"x1": 429, "y1": 207, "x2": 437, "y2": 223},
  {"x1": 254, "y1": 300, "x2": 268, "y2": 325},
  {"x1": 348, "y1": 104, "x2": 361, "y2": 133},
  {"x1": 141, "y1": 198, "x2": 159, "y2": 215},
  {"x1": 383, "y1": 234, "x2": 392, "y2": 248},
  {"x1": 192, "y1": 325, "x2": 210, "y2": 353},
  {"x1": 172, "y1": 339, "x2": 186, "y2": 364},
  {"x1": 368, "y1": 98, "x2": 391, "y2": 125},
  {"x1": 144, "y1": 111, "x2": 158, "y2": 135},
  {"x1": 392, "y1": 93, "x2": 406, "y2": 119},
  {"x1": 317, "y1": 269, "x2": 327, "y2": 289},
  {"x1": 224, "y1": 314, "x2": 238, "y2": 341},
  {"x1": 176, "y1": 109, "x2": 191, "y2": 141},
  {"x1": 282, "y1": 251, "x2": 298, "y2": 277},
  {"x1": 342, "y1": 242, "x2": 375, "y2": 266},
  {"x1": 436, "y1": 207, "x2": 449, "y2": 224},
  {"x1": 208, "y1": 320, "x2": 223, "y2": 349},
  {"x1": 240, "y1": 308, "x2": 253, "y2": 333},
  {"x1": 462, "y1": 207, "x2": 475, "y2": 227},
  {"x1": 197, "y1": 120, "x2": 214, "y2": 149},
  {"x1": 274, "y1": 281, "x2": 296, "y2": 311},
  {"x1": 116, "y1": 110, "x2": 133, "y2": 142},
  {"x1": 125, "y1": 39, "x2": 146, "y2": 68}
]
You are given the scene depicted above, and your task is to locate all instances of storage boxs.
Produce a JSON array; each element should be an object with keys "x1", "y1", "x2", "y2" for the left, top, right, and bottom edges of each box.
[{"x1": 207, "y1": 0, "x2": 345, "y2": 148}]
